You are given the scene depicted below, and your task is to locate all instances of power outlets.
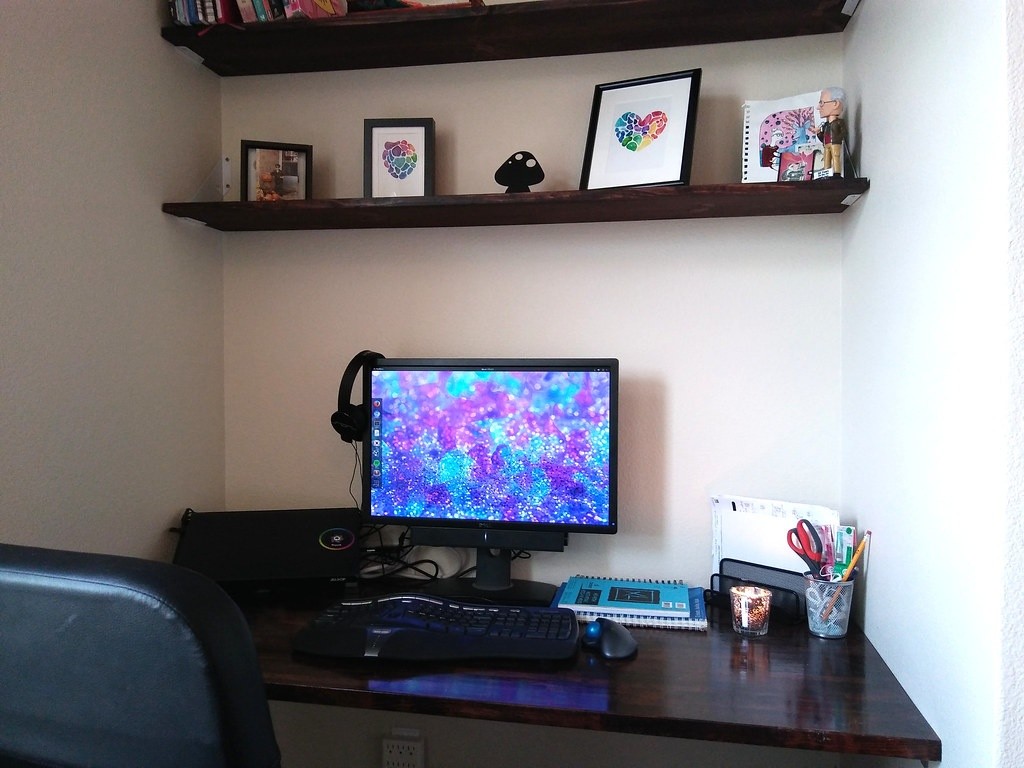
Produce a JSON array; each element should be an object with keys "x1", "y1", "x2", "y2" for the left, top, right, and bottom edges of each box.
[{"x1": 382, "y1": 736, "x2": 425, "y2": 768}]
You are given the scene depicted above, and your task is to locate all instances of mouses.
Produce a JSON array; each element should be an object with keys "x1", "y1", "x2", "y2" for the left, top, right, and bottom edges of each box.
[{"x1": 582, "y1": 617, "x2": 637, "y2": 662}]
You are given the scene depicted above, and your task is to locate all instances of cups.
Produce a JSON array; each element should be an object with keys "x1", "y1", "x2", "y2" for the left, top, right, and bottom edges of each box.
[{"x1": 729, "y1": 585, "x2": 772, "y2": 636}]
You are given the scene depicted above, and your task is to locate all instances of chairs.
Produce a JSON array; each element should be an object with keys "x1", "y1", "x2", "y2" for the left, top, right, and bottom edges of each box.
[{"x1": 0, "y1": 542, "x2": 283, "y2": 768}]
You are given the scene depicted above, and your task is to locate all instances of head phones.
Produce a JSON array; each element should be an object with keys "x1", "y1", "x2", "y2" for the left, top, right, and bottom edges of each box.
[{"x1": 331, "y1": 350, "x2": 386, "y2": 443}]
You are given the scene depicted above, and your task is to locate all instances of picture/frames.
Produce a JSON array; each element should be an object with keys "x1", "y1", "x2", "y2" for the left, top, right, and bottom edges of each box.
[
  {"x1": 579, "y1": 68, "x2": 703, "y2": 185},
  {"x1": 239, "y1": 139, "x2": 313, "y2": 200},
  {"x1": 362, "y1": 118, "x2": 436, "y2": 196}
]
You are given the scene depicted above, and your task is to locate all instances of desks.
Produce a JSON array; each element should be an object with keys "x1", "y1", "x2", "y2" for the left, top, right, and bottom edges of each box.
[{"x1": 243, "y1": 586, "x2": 942, "y2": 768}]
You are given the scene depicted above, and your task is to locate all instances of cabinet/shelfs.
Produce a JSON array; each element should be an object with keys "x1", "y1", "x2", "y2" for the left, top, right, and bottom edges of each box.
[{"x1": 160, "y1": 0, "x2": 870, "y2": 230}]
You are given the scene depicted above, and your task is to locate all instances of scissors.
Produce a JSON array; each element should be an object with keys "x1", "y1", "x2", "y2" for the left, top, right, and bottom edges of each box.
[{"x1": 786, "y1": 517, "x2": 845, "y2": 613}]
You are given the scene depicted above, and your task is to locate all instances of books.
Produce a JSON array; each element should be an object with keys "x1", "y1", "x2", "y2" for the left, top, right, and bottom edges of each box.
[
  {"x1": 169, "y1": 0, "x2": 348, "y2": 24},
  {"x1": 550, "y1": 574, "x2": 707, "y2": 631}
]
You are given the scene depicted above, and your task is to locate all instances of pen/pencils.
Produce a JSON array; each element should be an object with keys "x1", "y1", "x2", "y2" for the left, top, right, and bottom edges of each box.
[{"x1": 821, "y1": 528, "x2": 873, "y2": 622}]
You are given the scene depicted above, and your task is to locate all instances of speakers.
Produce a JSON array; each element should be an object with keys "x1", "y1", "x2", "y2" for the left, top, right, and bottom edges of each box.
[{"x1": 176, "y1": 507, "x2": 362, "y2": 611}]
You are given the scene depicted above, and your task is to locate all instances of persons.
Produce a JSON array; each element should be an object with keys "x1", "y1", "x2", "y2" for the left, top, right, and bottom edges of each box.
[
  {"x1": 817, "y1": 87, "x2": 848, "y2": 176},
  {"x1": 270, "y1": 164, "x2": 285, "y2": 197}
]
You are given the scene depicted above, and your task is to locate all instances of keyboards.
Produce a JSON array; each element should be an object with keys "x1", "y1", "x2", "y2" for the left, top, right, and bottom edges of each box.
[{"x1": 290, "y1": 592, "x2": 582, "y2": 676}]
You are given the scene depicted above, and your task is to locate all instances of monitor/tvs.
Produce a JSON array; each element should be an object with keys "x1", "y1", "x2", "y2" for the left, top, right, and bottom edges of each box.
[{"x1": 362, "y1": 357, "x2": 620, "y2": 609}]
